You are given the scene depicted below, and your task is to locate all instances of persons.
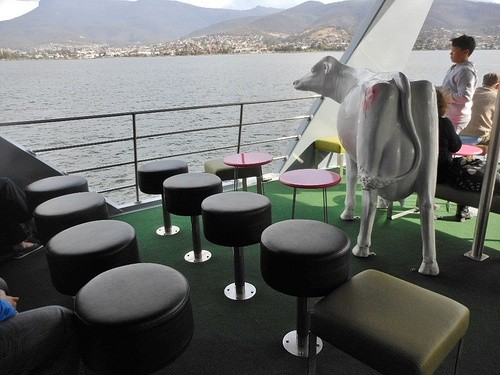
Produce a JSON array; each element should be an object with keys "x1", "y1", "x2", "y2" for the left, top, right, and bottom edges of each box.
[
  {"x1": 436, "y1": 89, "x2": 477, "y2": 222},
  {"x1": 459, "y1": 73, "x2": 500, "y2": 145},
  {"x1": 441, "y1": 35, "x2": 477, "y2": 134},
  {"x1": 0, "y1": 177, "x2": 43, "y2": 259},
  {"x1": 0, "y1": 278, "x2": 79, "y2": 375}
]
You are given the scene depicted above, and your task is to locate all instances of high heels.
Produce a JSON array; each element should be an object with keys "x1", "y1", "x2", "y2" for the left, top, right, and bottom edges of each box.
[{"x1": 456, "y1": 204, "x2": 472, "y2": 222}]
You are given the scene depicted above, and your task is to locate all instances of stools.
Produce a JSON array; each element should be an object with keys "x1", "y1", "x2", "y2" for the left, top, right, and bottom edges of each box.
[
  {"x1": 163, "y1": 172, "x2": 223, "y2": 262},
  {"x1": 46, "y1": 219, "x2": 139, "y2": 294},
  {"x1": 205, "y1": 159, "x2": 263, "y2": 193},
  {"x1": 308, "y1": 269, "x2": 470, "y2": 375},
  {"x1": 137, "y1": 159, "x2": 188, "y2": 235},
  {"x1": 262, "y1": 219, "x2": 351, "y2": 357},
  {"x1": 32, "y1": 191, "x2": 110, "y2": 246},
  {"x1": 24, "y1": 175, "x2": 90, "y2": 215},
  {"x1": 280, "y1": 167, "x2": 342, "y2": 223},
  {"x1": 456, "y1": 145, "x2": 483, "y2": 156},
  {"x1": 201, "y1": 191, "x2": 272, "y2": 301},
  {"x1": 224, "y1": 152, "x2": 271, "y2": 191},
  {"x1": 314, "y1": 138, "x2": 347, "y2": 179},
  {"x1": 71, "y1": 261, "x2": 195, "y2": 375}
]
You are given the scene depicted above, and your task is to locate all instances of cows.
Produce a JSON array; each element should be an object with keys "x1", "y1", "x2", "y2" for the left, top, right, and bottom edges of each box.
[{"x1": 292, "y1": 56, "x2": 440, "y2": 276}]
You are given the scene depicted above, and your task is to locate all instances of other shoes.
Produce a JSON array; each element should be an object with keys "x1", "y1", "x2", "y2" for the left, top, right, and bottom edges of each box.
[{"x1": 14, "y1": 243, "x2": 43, "y2": 259}]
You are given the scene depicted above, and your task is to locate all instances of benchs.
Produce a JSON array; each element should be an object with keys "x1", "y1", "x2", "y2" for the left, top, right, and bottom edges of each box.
[{"x1": 386, "y1": 177, "x2": 500, "y2": 221}]
